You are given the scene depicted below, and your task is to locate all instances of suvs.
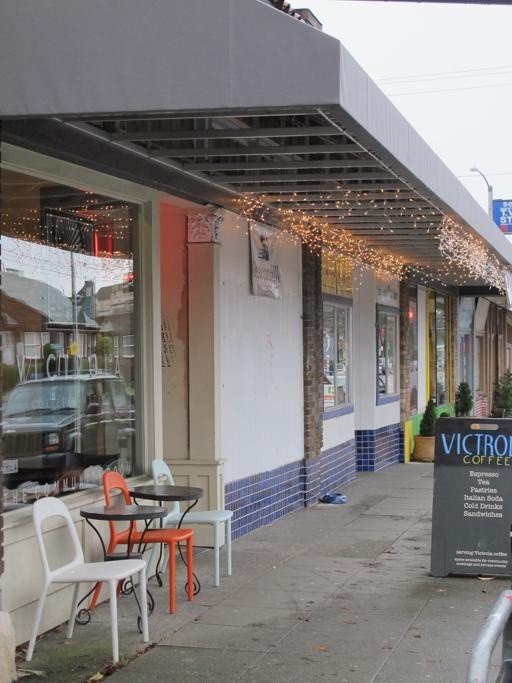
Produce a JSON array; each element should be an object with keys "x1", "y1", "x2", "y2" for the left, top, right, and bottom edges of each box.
[{"x1": 0, "y1": 370, "x2": 138, "y2": 489}]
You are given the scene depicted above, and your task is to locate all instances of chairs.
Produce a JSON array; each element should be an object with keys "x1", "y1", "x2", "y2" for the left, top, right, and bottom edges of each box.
[
  {"x1": 25, "y1": 496, "x2": 148, "y2": 664},
  {"x1": 151, "y1": 458, "x2": 233, "y2": 587},
  {"x1": 90, "y1": 472, "x2": 194, "y2": 615}
]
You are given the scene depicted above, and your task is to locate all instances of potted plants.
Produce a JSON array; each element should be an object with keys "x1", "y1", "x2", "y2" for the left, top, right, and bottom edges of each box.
[{"x1": 413, "y1": 399, "x2": 436, "y2": 462}]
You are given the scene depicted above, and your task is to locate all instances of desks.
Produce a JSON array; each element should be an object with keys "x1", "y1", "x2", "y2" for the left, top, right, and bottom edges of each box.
[{"x1": 76, "y1": 504, "x2": 168, "y2": 634}]
